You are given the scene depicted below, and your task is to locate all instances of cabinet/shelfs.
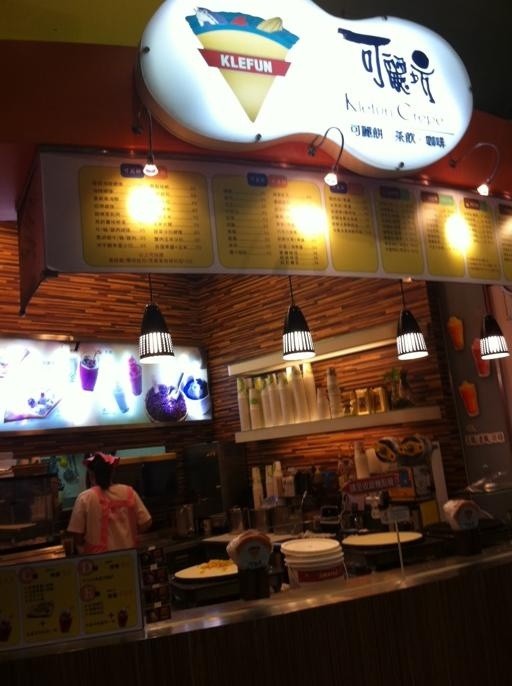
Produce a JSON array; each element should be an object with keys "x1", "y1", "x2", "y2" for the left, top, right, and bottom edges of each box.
[{"x1": 226, "y1": 318, "x2": 442, "y2": 444}]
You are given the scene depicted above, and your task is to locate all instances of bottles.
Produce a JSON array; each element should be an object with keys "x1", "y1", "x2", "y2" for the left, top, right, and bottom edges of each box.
[{"x1": 382, "y1": 369, "x2": 414, "y2": 410}]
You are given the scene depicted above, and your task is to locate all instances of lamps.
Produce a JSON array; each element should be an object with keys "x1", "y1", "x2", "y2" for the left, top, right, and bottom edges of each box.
[
  {"x1": 393, "y1": 277, "x2": 432, "y2": 362},
  {"x1": 449, "y1": 143, "x2": 504, "y2": 197},
  {"x1": 136, "y1": 273, "x2": 177, "y2": 363},
  {"x1": 282, "y1": 273, "x2": 315, "y2": 362},
  {"x1": 477, "y1": 286, "x2": 509, "y2": 361},
  {"x1": 311, "y1": 127, "x2": 345, "y2": 188},
  {"x1": 130, "y1": 103, "x2": 158, "y2": 179}
]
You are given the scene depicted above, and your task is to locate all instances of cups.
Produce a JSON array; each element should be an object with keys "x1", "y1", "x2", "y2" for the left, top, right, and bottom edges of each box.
[
  {"x1": 118, "y1": 614, "x2": 128, "y2": 627},
  {"x1": 200, "y1": 517, "x2": 212, "y2": 532},
  {"x1": 459, "y1": 384, "x2": 481, "y2": 416},
  {"x1": 446, "y1": 321, "x2": 465, "y2": 350},
  {"x1": 80, "y1": 365, "x2": 97, "y2": 390},
  {"x1": 0, "y1": 625, "x2": 12, "y2": 641},
  {"x1": 252, "y1": 459, "x2": 295, "y2": 509},
  {"x1": 472, "y1": 339, "x2": 490, "y2": 377},
  {"x1": 235, "y1": 362, "x2": 343, "y2": 431},
  {"x1": 131, "y1": 367, "x2": 142, "y2": 396},
  {"x1": 59, "y1": 616, "x2": 72, "y2": 632},
  {"x1": 353, "y1": 441, "x2": 370, "y2": 481}
]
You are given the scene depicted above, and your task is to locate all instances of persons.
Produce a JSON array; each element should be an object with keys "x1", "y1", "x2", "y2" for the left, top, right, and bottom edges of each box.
[{"x1": 66, "y1": 452, "x2": 154, "y2": 557}]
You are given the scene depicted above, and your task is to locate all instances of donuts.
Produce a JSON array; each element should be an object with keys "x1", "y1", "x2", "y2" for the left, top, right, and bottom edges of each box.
[{"x1": 143, "y1": 384, "x2": 187, "y2": 423}]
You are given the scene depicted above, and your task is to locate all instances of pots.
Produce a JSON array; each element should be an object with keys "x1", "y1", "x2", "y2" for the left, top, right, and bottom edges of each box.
[{"x1": 176, "y1": 503, "x2": 196, "y2": 538}]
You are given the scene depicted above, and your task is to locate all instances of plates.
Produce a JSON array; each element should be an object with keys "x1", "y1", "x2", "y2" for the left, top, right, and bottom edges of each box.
[{"x1": 282, "y1": 538, "x2": 345, "y2": 568}]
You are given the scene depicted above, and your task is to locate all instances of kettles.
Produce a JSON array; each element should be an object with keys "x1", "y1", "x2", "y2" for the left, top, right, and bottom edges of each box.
[{"x1": 229, "y1": 503, "x2": 251, "y2": 534}]
